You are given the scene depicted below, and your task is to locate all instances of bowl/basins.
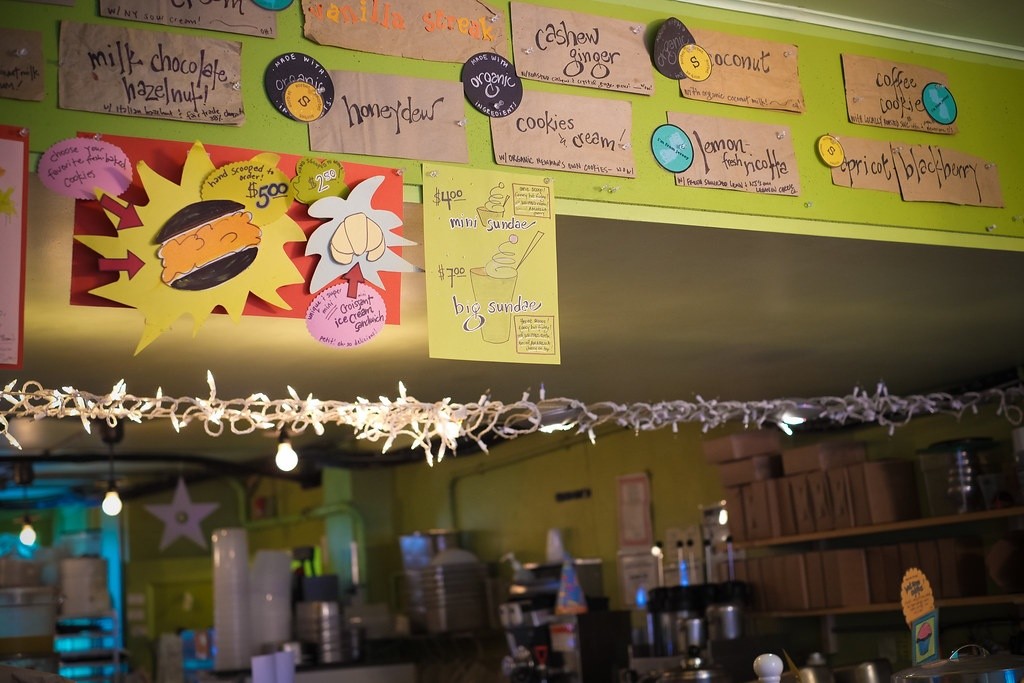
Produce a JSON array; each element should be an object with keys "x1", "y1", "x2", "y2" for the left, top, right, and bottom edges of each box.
[{"x1": 0, "y1": 559, "x2": 39, "y2": 590}]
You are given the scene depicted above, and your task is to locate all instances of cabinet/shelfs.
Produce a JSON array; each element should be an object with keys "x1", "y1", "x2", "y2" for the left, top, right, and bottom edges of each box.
[{"x1": 716, "y1": 506, "x2": 1024, "y2": 619}]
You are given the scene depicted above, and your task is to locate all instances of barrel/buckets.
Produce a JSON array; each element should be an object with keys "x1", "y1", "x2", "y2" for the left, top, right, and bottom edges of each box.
[
  {"x1": 60, "y1": 556, "x2": 110, "y2": 618},
  {"x1": 415, "y1": 561, "x2": 493, "y2": 633},
  {"x1": 396, "y1": 527, "x2": 462, "y2": 615}
]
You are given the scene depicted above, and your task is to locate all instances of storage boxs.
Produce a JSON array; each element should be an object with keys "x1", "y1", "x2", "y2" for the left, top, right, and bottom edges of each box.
[
  {"x1": 791, "y1": 468, "x2": 815, "y2": 534},
  {"x1": 784, "y1": 553, "x2": 809, "y2": 611},
  {"x1": 918, "y1": 540, "x2": 943, "y2": 601},
  {"x1": 882, "y1": 545, "x2": 901, "y2": 602},
  {"x1": 863, "y1": 461, "x2": 922, "y2": 525},
  {"x1": 777, "y1": 470, "x2": 798, "y2": 535},
  {"x1": 866, "y1": 547, "x2": 887, "y2": 604},
  {"x1": 939, "y1": 535, "x2": 986, "y2": 600},
  {"x1": 821, "y1": 551, "x2": 869, "y2": 608},
  {"x1": 760, "y1": 556, "x2": 789, "y2": 611},
  {"x1": 804, "y1": 552, "x2": 826, "y2": 610},
  {"x1": 781, "y1": 441, "x2": 867, "y2": 475},
  {"x1": 727, "y1": 556, "x2": 765, "y2": 613},
  {"x1": 703, "y1": 429, "x2": 781, "y2": 464},
  {"x1": 848, "y1": 458, "x2": 897, "y2": 526},
  {"x1": 728, "y1": 479, "x2": 783, "y2": 542},
  {"x1": 807, "y1": 471, "x2": 835, "y2": 531},
  {"x1": 826, "y1": 465, "x2": 855, "y2": 530},
  {"x1": 900, "y1": 543, "x2": 919, "y2": 602},
  {"x1": 720, "y1": 455, "x2": 782, "y2": 487}
]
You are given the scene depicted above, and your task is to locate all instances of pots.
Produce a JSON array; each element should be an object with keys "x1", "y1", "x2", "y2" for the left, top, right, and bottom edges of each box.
[{"x1": 0, "y1": 584, "x2": 59, "y2": 654}]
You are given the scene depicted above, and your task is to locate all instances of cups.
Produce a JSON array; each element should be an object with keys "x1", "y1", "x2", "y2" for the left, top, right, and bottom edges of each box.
[
  {"x1": 249, "y1": 654, "x2": 274, "y2": 683},
  {"x1": 707, "y1": 604, "x2": 737, "y2": 647},
  {"x1": 298, "y1": 575, "x2": 339, "y2": 601},
  {"x1": 295, "y1": 600, "x2": 343, "y2": 667},
  {"x1": 273, "y1": 653, "x2": 295, "y2": 683},
  {"x1": 675, "y1": 616, "x2": 700, "y2": 653},
  {"x1": 283, "y1": 641, "x2": 302, "y2": 665}
]
